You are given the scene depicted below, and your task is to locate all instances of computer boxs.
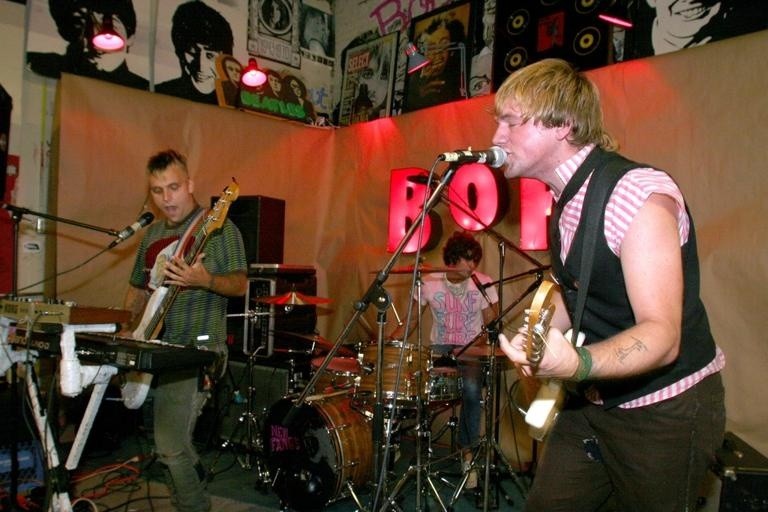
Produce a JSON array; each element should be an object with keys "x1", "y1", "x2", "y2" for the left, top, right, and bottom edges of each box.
[{"x1": 243, "y1": 275, "x2": 317, "y2": 359}]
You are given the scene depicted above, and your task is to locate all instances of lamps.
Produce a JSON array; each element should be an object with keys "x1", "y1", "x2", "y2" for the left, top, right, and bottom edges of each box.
[
  {"x1": 599, "y1": 0, "x2": 635, "y2": 28},
  {"x1": 88, "y1": 12, "x2": 123, "y2": 52},
  {"x1": 242, "y1": 58, "x2": 266, "y2": 88}
]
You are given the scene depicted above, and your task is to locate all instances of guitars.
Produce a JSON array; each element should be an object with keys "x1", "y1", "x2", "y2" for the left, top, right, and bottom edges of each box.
[
  {"x1": 116, "y1": 177, "x2": 240, "y2": 410},
  {"x1": 523, "y1": 281, "x2": 565, "y2": 443}
]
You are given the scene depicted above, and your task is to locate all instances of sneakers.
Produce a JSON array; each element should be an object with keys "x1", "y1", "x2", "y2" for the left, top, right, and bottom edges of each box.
[{"x1": 459, "y1": 461, "x2": 478, "y2": 490}]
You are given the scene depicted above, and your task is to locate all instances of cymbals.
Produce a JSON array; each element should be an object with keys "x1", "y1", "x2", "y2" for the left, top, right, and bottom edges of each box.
[
  {"x1": 269, "y1": 328, "x2": 354, "y2": 355},
  {"x1": 250, "y1": 290, "x2": 336, "y2": 305},
  {"x1": 454, "y1": 347, "x2": 507, "y2": 358},
  {"x1": 369, "y1": 261, "x2": 472, "y2": 274}
]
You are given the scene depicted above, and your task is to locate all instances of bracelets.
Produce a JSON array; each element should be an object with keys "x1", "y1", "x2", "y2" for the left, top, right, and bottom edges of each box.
[{"x1": 569, "y1": 346, "x2": 592, "y2": 387}]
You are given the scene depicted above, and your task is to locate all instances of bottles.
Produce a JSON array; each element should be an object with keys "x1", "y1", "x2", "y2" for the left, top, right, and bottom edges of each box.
[{"x1": 0, "y1": 449, "x2": 33, "y2": 473}]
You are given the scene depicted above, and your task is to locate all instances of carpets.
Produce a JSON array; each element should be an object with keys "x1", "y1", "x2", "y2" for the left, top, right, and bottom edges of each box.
[{"x1": 83, "y1": 434, "x2": 532, "y2": 511}]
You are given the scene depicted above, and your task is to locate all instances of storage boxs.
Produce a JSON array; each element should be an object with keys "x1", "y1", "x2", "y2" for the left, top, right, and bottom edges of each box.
[{"x1": 695, "y1": 431, "x2": 768, "y2": 512}]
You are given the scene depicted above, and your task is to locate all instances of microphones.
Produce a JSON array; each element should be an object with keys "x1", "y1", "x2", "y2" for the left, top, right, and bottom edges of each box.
[
  {"x1": 438, "y1": 145, "x2": 508, "y2": 170},
  {"x1": 110, "y1": 213, "x2": 154, "y2": 247},
  {"x1": 470, "y1": 273, "x2": 492, "y2": 307}
]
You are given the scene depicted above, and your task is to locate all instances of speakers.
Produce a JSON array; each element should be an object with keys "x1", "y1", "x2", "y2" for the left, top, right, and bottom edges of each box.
[
  {"x1": 204, "y1": 357, "x2": 312, "y2": 451},
  {"x1": 211, "y1": 194, "x2": 285, "y2": 356}
]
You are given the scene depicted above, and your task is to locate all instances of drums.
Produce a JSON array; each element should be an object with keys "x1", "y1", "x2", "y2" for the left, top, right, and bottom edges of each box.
[
  {"x1": 255, "y1": 388, "x2": 374, "y2": 511},
  {"x1": 425, "y1": 345, "x2": 464, "y2": 368},
  {"x1": 428, "y1": 368, "x2": 462, "y2": 402},
  {"x1": 354, "y1": 340, "x2": 430, "y2": 403},
  {"x1": 312, "y1": 356, "x2": 362, "y2": 392}
]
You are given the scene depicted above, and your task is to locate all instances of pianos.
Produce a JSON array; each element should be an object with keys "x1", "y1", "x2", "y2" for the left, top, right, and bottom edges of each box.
[{"x1": 7, "y1": 325, "x2": 220, "y2": 372}]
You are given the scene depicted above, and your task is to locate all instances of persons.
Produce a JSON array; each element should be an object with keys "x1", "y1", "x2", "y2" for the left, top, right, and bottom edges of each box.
[
  {"x1": 155, "y1": 0, "x2": 234, "y2": 106},
  {"x1": 407, "y1": 16, "x2": 462, "y2": 112},
  {"x1": 387, "y1": 234, "x2": 500, "y2": 488},
  {"x1": 124, "y1": 149, "x2": 249, "y2": 512},
  {"x1": 26, "y1": 0, "x2": 150, "y2": 91},
  {"x1": 624, "y1": 0, "x2": 723, "y2": 61},
  {"x1": 492, "y1": 57, "x2": 725, "y2": 512},
  {"x1": 222, "y1": 57, "x2": 313, "y2": 124}
]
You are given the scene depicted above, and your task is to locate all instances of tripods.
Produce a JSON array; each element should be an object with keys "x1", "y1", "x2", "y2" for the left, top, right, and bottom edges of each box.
[
  {"x1": 445, "y1": 328, "x2": 526, "y2": 511},
  {"x1": 379, "y1": 258, "x2": 469, "y2": 511},
  {"x1": 208, "y1": 321, "x2": 265, "y2": 491}
]
